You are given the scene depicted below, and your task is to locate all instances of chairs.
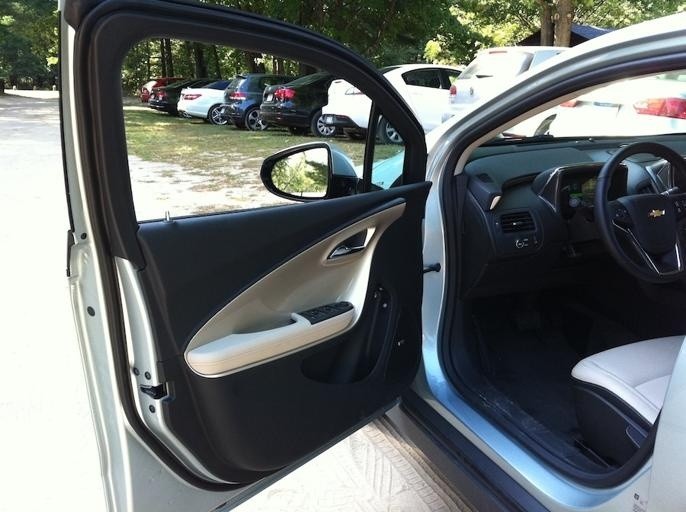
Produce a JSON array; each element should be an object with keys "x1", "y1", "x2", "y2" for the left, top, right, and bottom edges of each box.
[{"x1": 568, "y1": 333, "x2": 686, "y2": 468}]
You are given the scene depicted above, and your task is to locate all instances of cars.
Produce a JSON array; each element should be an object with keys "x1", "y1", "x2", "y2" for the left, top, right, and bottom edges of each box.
[
  {"x1": 178, "y1": 78, "x2": 226, "y2": 125},
  {"x1": 147, "y1": 80, "x2": 194, "y2": 116},
  {"x1": 322, "y1": 63, "x2": 467, "y2": 141},
  {"x1": 549, "y1": 69, "x2": 686, "y2": 137},
  {"x1": 51, "y1": 0, "x2": 686, "y2": 512},
  {"x1": 260, "y1": 71, "x2": 342, "y2": 135},
  {"x1": 140, "y1": 77, "x2": 184, "y2": 104}
]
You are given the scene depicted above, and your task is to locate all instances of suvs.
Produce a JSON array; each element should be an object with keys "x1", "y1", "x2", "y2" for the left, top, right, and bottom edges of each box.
[
  {"x1": 442, "y1": 44, "x2": 570, "y2": 136},
  {"x1": 222, "y1": 71, "x2": 290, "y2": 132}
]
